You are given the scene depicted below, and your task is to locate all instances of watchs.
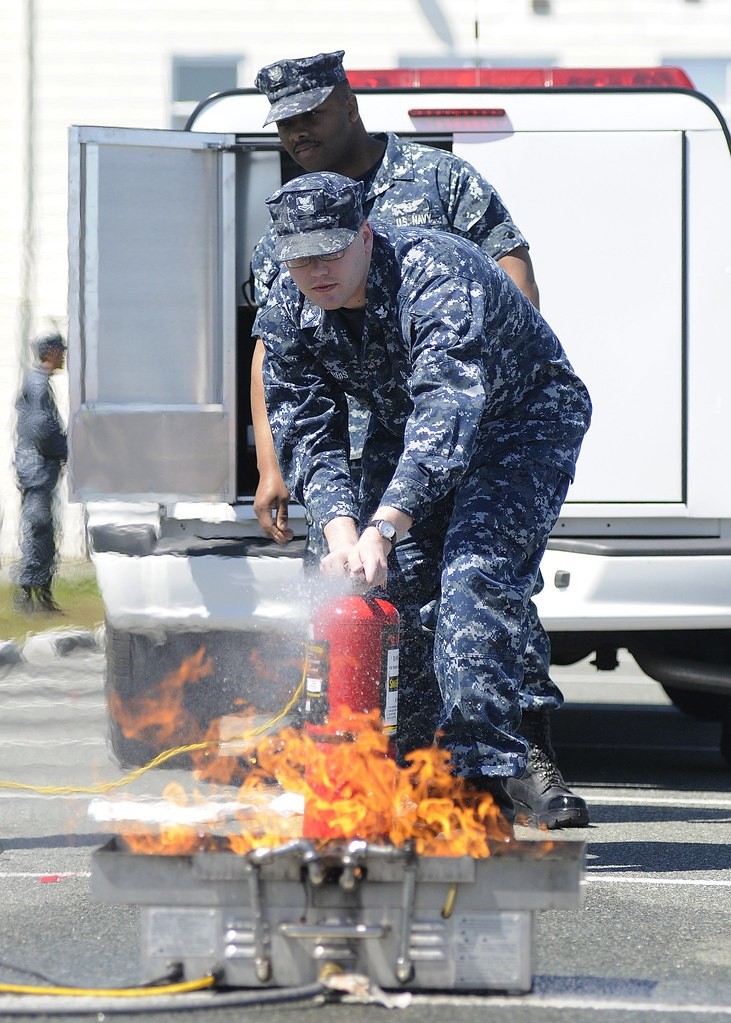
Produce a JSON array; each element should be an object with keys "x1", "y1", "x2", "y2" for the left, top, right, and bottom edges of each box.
[{"x1": 365, "y1": 520, "x2": 397, "y2": 549}]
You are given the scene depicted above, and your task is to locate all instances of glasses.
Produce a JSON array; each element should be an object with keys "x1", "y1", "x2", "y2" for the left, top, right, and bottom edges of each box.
[{"x1": 285, "y1": 250, "x2": 345, "y2": 269}]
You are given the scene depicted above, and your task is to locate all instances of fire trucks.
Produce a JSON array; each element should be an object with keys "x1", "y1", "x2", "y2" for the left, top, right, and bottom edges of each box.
[{"x1": 67, "y1": 63, "x2": 731, "y2": 768}]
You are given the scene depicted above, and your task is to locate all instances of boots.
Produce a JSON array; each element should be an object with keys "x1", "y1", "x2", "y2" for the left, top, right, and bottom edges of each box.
[
  {"x1": 32, "y1": 587, "x2": 64, "y2": 616},
  {"x1": 506, "y1": 710, "x2": 591, "y2": 829},
  {"x1": 8, "y1": 588, "x2": 32, "y2": 615}
]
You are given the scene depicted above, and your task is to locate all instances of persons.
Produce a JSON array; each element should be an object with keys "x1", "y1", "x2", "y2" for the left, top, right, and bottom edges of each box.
[
  {"x1": 258, "y1": 170, "x2": 598, "y2": 829},
  {"x1": 248, "y1": 50, "x2": 541, "y2": 764},
  {"x1": 14, "y1": 331, "x2": 75, "y2": 613}
]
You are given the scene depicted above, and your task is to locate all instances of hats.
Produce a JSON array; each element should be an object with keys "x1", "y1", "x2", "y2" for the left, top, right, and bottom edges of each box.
[
  {"x1": 253, "y1": 50, "x2": 349, "y2": 129},
  {"x1": 264, "y1": 171, "x2": 365, "y2": 262},
  {"x1": 36, "y1": 334, "x2": 68, "y2": 357}
]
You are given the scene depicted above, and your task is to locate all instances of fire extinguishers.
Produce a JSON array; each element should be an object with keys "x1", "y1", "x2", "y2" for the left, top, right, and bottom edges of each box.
[{"x1": 303, "y1": 561, "x2": 400, "y2": 840}]
